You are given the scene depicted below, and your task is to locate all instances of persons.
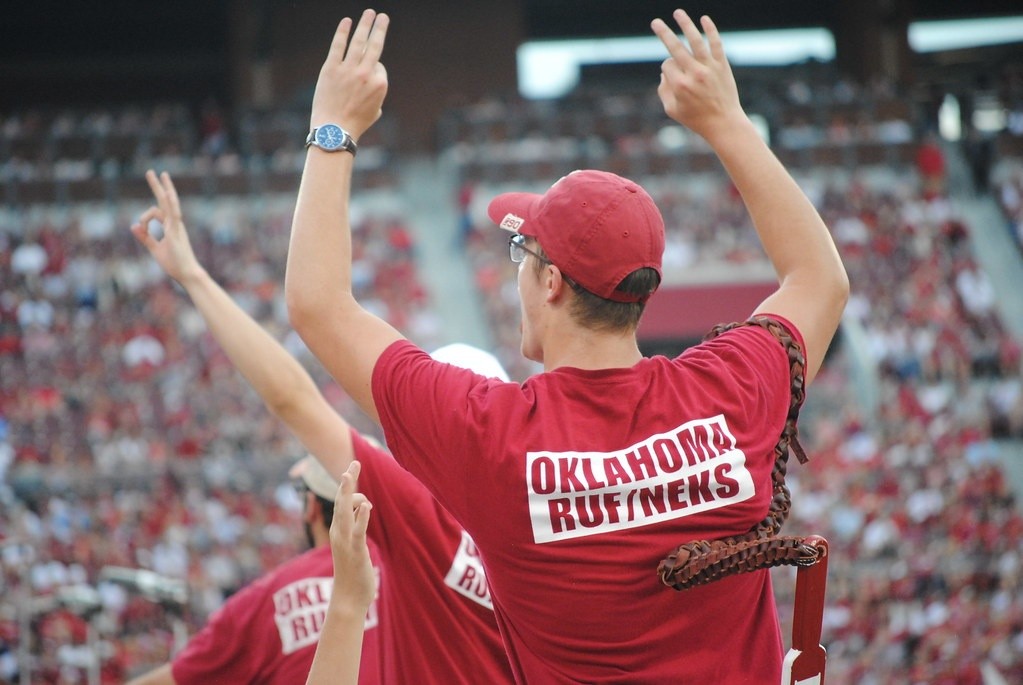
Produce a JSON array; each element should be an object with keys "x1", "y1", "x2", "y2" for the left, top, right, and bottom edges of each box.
[
  {"x1": 131, "y1": 166, "x2": 515, "y2": 684},
  {"x1": 0, "y1": 1, "x2": 1022, "y2": 684},
  {"x1": 127, "y1": 432, "x2": 381, "y2": 685},
  {"x1": 306, "y1": 458, "x2": 374, "y2": 684},
  {"x1": 285, "y1": 9, "x2": 849, "y2": 685}
]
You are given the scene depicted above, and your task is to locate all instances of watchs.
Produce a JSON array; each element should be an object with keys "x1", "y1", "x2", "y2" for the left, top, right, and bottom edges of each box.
[{"x1": 305, "y1": 124, "x2": 358, "y2": 156}]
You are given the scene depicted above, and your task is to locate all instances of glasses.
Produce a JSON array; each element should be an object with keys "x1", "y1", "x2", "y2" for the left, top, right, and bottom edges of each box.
[
  {"x1": 294, "y1": 485, "x2": 311, "y2": 501},
  {"x1": 508, "y1": 232, "x2": 577, "y2": 290}
]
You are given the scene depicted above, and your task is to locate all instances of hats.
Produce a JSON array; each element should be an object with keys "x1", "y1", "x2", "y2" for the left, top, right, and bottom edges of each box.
[
  {"x1": 487, "y1": 169, "x2": 665, "y2": 303},
  {"x1": 289, "y1": 432, "x2": 382, "y2": 503},
  {"x1": 431, "y1": 344, "x2": 511, "y2": 384}
]
